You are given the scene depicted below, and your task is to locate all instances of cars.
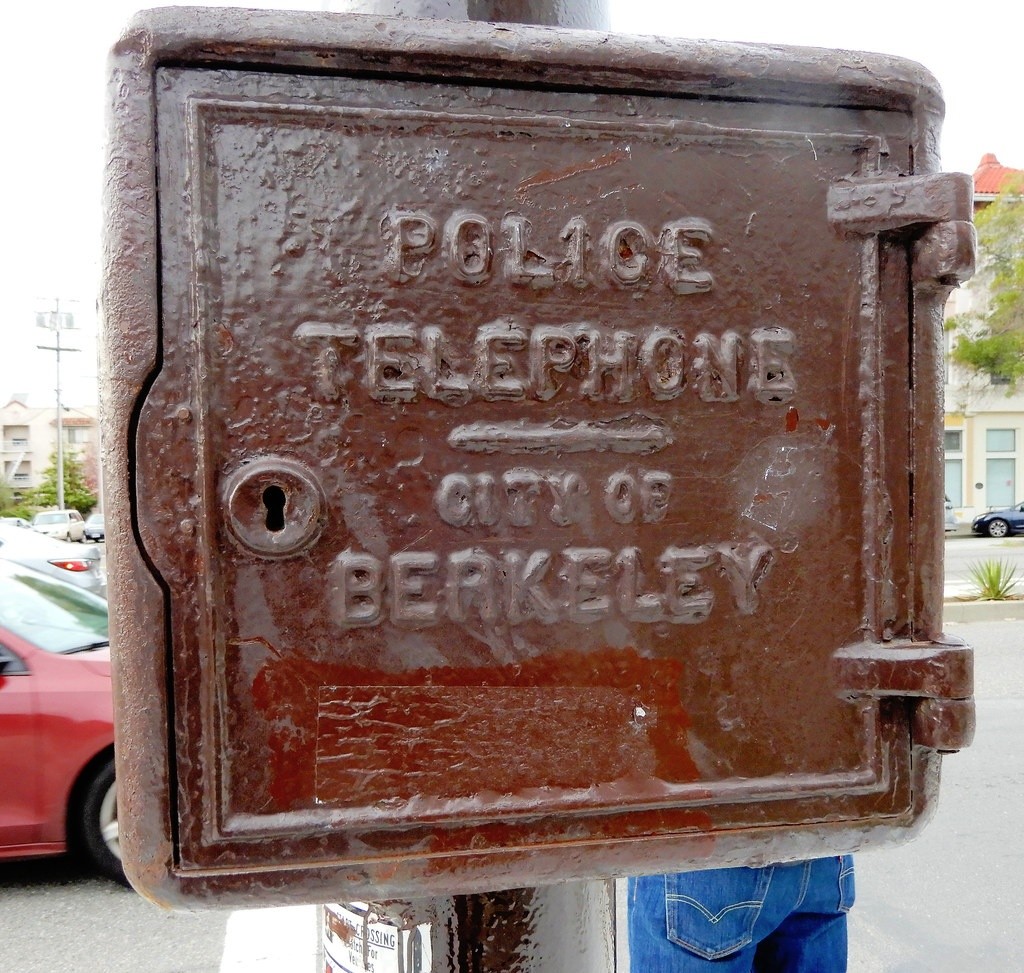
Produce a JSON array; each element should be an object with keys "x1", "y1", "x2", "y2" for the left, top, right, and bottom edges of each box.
[
  {"x1": 0, "y1": 510, "x2": 135, "y2": 897},
  {"x1": 970, "y1": 501, "x2": 1024, "y2": 537},
  {"x1": 944, "y1": 493, "x2": 958, "y2": 533}
]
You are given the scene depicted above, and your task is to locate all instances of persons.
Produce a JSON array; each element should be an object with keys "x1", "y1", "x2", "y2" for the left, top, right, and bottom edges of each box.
[{"x1": 627, "y1": 852, "x2": 854, "y2": 973}]
